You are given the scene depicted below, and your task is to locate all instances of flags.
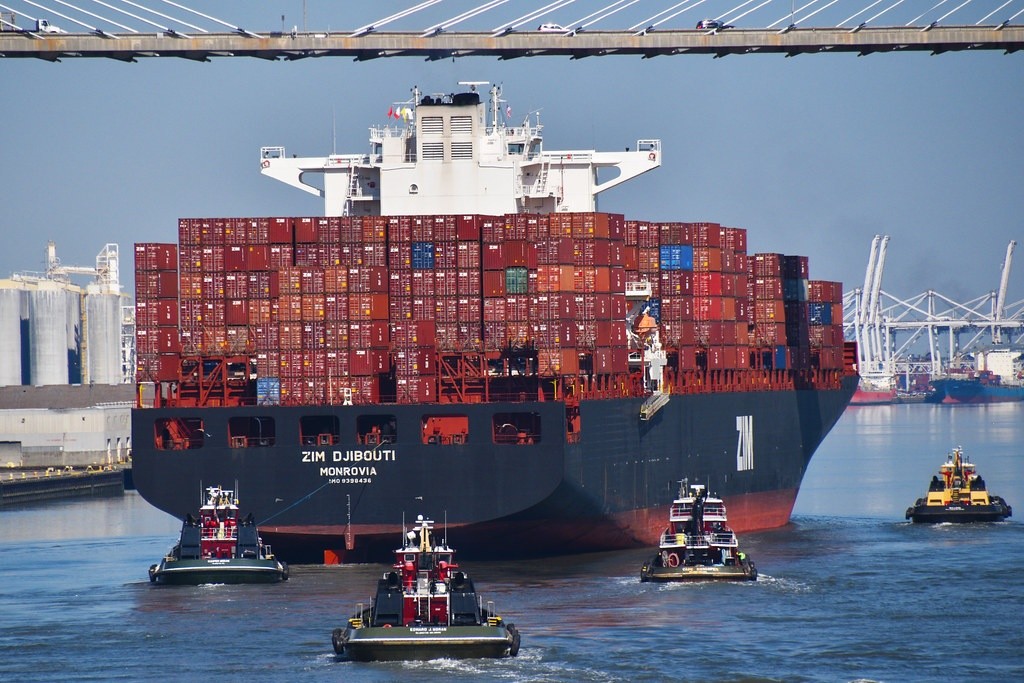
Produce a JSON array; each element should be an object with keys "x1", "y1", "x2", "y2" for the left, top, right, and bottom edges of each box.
[
  {"x1": 386, "y1": 107, "x2": 392, "y2": 118},
  {"x1": 506, "y1": 107, "x2": 512, "y2": 118},
  {"x1": 400, "y1": 108, "x2": 407, "y2": 123},
  {"x1": 393, "y1": 106, "x2": 400, "y2": 119}
]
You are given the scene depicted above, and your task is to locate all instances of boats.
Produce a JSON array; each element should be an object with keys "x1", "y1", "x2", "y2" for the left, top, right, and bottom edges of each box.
[
  {"x1": 331, "y1": 509, "x2": 521, "y2": 658},
  {"x1": 148, "y1": 477, "x2": 289, "y2": 586},
  {"x1": 640, "y1": 474, "x2": 758, "y2": 582},
  {"x1": 904, "y1": 444, "x2": 1013, "y2": 523},
  {"x1": 850, "y1": 377, "x2": 895, "y2": 404},
  {"x1": 924, "y1": 366, "x2": 1024, "y2": 404}
]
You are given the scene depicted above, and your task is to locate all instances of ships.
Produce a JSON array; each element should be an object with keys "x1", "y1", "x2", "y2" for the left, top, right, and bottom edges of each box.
[{"x1": 130, "y1": 79, "x2": 862, "y2": 565}]
